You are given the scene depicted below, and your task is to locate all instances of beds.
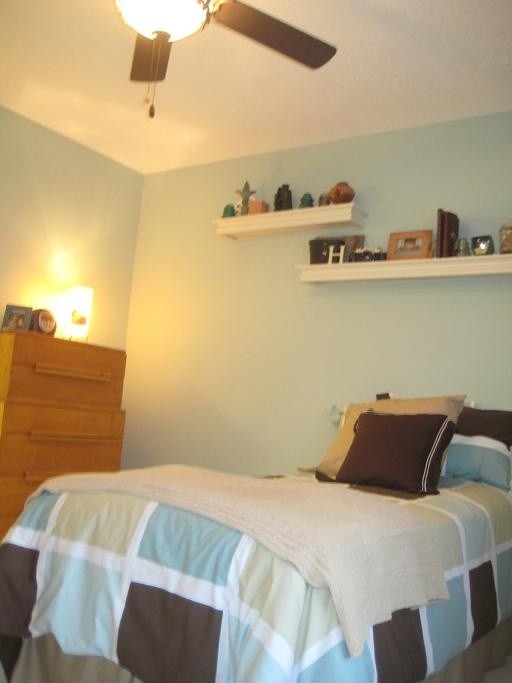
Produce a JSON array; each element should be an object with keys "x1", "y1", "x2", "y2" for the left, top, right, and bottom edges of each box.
[{"x1": 0, "y1": 407, "x2": 512, "y2": 683}]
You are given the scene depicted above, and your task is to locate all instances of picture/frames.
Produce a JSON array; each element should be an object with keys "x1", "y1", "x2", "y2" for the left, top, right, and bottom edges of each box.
[
  {"x1": 387, "y1": 230, "x2": 432, "y2": 259},
  {"x1": 1, "y1": 304, "x2": 33, "y2": 333}
]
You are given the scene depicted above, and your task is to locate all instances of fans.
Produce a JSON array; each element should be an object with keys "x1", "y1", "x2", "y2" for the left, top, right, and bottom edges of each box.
[{"x1": 130, "y1": 0, "x2": 338, "y2": 83}]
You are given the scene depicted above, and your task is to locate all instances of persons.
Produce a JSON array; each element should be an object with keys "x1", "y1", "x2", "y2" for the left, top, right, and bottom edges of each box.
[{"x1": 398, "y1": 239, "x2": 420, "y2": 252}]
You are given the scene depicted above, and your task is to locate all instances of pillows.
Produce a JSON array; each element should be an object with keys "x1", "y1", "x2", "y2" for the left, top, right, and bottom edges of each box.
[
  {"x1": 335, "y1": 412, "x2": 456, "y2": 496},
  {"x1": 320, "y1": 394, "x2": 466, "y2": 483}
]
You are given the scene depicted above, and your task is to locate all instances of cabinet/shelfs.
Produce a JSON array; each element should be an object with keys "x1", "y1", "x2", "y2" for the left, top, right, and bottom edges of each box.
[
  {"x1": 0, "y1": 331, "x2": 126, "y2": 543},
  {"x1": 297, "y1": 253, "x2": 511, "y2": 282},
  {"x1": 217, "y1": 202, "x2": 369, "y2": 241}
]
